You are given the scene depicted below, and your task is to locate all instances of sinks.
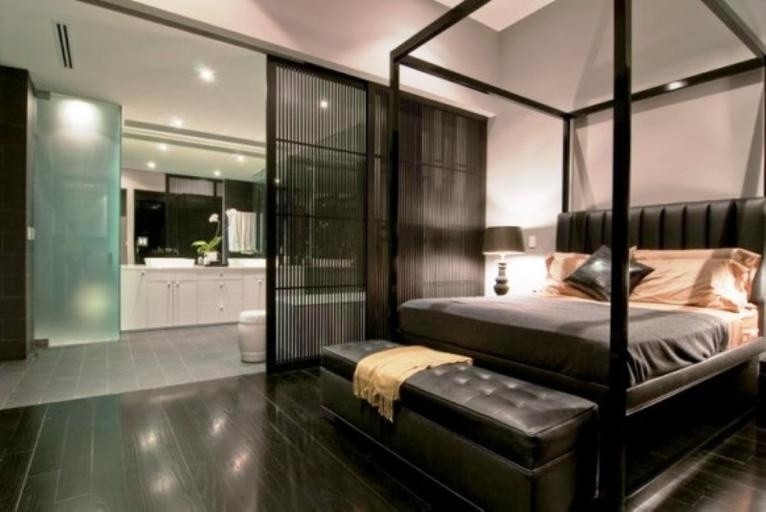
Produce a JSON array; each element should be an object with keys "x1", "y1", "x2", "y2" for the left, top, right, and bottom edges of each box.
[
  {"x1": 144, "y1": 255, "x2": 196, "y2": 268},
  {"x1": 226, "y1": 258, "x2": 266, "y2": 268}
]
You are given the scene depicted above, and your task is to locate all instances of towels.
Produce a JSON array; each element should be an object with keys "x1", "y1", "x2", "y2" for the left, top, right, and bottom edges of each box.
[
  {"x1": 351, "y1": 343, "x2": 474, "y2": 422},
  {"x1": 226, "y1": 208, "x2": 260, "y2": 258}
]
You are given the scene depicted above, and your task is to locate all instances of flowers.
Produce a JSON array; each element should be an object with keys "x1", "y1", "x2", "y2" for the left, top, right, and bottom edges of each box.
[{"x1": 192, "y1": 213, "x2": 224, "y2": 253}]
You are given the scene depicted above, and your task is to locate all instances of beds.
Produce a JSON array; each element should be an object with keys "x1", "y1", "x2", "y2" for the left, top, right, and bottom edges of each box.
[{"x1": 318, "y1": 0, "x2": 766, "y2": 512}]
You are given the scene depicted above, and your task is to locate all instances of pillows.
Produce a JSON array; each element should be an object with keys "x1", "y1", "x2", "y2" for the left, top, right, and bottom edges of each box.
[{"x1": 542, "y1": 242, "x2": 764, "y2": 315}]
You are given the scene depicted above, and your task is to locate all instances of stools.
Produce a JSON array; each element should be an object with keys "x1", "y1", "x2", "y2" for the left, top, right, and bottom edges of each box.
[{"x1": 238, "y1": 311, "x2": 267, "y2": 365}]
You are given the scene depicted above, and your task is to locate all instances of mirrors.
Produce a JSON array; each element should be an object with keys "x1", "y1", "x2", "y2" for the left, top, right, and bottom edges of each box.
[{"x1": 134, "y1": 188, "x2": 222, "y2": 265}]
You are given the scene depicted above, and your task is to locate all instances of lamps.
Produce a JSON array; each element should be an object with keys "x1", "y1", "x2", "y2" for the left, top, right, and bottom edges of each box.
[{"x1": 481, "y1": 224, "x2": 527, "y2": 296}]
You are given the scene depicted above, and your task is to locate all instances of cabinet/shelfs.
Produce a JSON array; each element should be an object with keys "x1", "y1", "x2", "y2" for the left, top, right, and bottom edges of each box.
[{"x1": 118, "y1": 264, "x2": 267, "y2": 333}]
